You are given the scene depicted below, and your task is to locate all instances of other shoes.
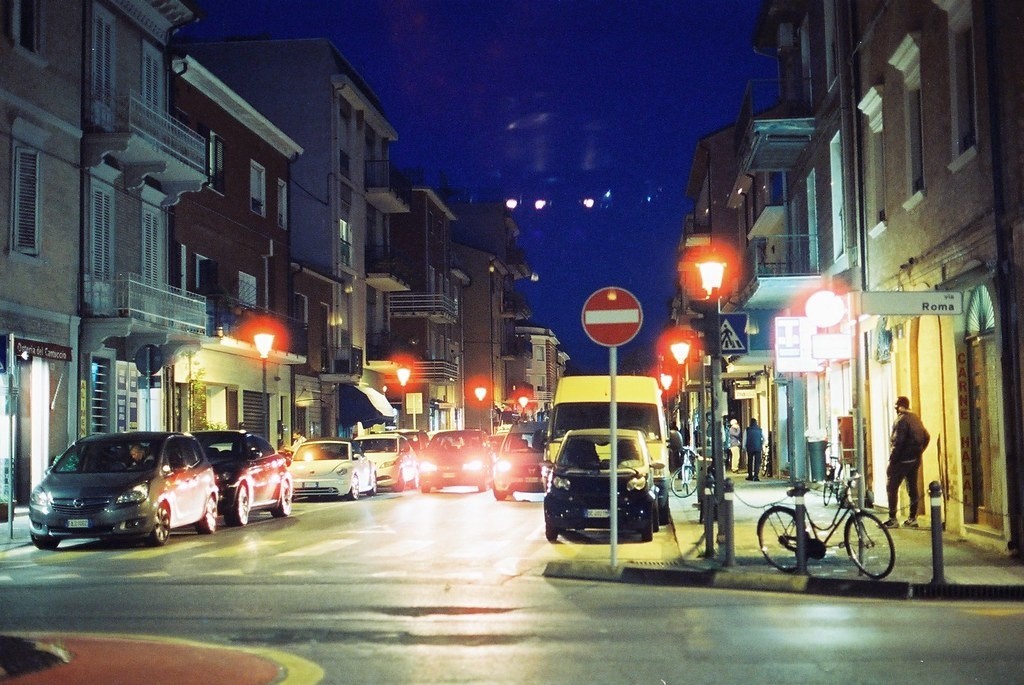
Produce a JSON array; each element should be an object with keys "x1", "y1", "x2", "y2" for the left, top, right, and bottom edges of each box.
[
  {"x1": 901, "y1": 516, "x2": 919, "y2": 529},
  {"x1": 753, "y1": 476, "x2": 760, "y2": 481},
  {"x1": 745, "y1": 476, "x2": 753, "y2": 481},
  {"x1": 875, "y1": 517, "x2": 899, "y2": 529}
]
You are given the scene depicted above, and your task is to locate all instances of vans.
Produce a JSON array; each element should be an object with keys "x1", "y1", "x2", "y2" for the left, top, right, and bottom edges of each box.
[
  {"x1": 490, "y1": 420, "x2": 548, "y2": 502},
  {"x1": 531, "y1": 376, "x2": 683, "y2": 527},
  {"x1": 539, "y1": 427, "x2": 665, "y2": 543}
]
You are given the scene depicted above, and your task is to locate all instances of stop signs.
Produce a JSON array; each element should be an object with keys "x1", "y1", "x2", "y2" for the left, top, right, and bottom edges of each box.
[{"x1": 582, "y1": 286, "x2": 643, "y2": 348}]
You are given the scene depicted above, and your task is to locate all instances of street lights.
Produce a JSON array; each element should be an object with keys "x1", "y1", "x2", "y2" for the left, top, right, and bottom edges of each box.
[
  {"x1": 660, "y1": 373, "x2": 673, "y2": 439},
  {"x1": 694, "y1": 260, "x2": 728, "y2": 503},
  {"x1": 396, "y1": 368, "x2": 410, "y2": 429},
  {"x1": 474, "y1": 388, "x2": 487, "y2": 432},
  {"x1": 253, "y1": 333, "x2": 275, "y2": 443},
  {"x1": 518, "y1": 396, "x2": 529, "y2": 423},
  {"x1": 669, "y1": 341, "x2": 690, "y2": 447}
]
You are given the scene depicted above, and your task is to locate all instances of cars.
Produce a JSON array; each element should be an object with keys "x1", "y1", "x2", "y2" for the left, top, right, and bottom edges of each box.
[
  {"x1": 354, "y1": 433, "x2": 418, "y2": 493},
  {"x1": 417, "y1": 428, "x2": 492, "y2": 495},
  {"x1": 379, "y1": 428, "x2": 465, "y2": 455},
  {"x1": 489, "y1": 423, "x2": 513, "y2": 459},
  {"x1": 287, "y1": 437, "x2": 377, "y2": 503},
  {"x1": 188, "y1": 429, "x2": 293, "y2": 527},
  {"x1": 27, "y1": 430, "x2": 219, "y2": 550}
]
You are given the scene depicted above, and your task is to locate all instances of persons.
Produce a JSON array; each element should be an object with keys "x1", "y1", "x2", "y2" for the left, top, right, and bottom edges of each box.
[
  {"x1": 492, "y1": 402, "x2": 553, "y2": 424},
  {"x1": 669, "y1": 424, "x2": 699, "y2": 479},
  {"x1": 706, "y1": 412, "x2": 712, "y2": 445},
  {"x1": 291, "y1": 429, "x2": 307, "y2": 447},
  {"x1": 742, "y1": 418, "x2": 764, "y2": 481},
  {"x1": 127, "y1": 445, "x2": 152, "y2": 470},
  {"x1": 876, "y1": 396, "x2": 930, "y2": 528},
  {"x1": 729, "y1": 419, "x2": 743, "y2": 474}
]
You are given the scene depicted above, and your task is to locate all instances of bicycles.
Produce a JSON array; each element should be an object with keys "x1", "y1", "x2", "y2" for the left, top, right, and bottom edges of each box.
[
  {"x1": 755, "y1": 471, "x2": 896, "y2": 579},
  {"x1": 822, "y1": 456, "x2": 850, "y2": 506},
  {"x1": 670, "y1": 445, "x2": 700, "y2": 498}
]
